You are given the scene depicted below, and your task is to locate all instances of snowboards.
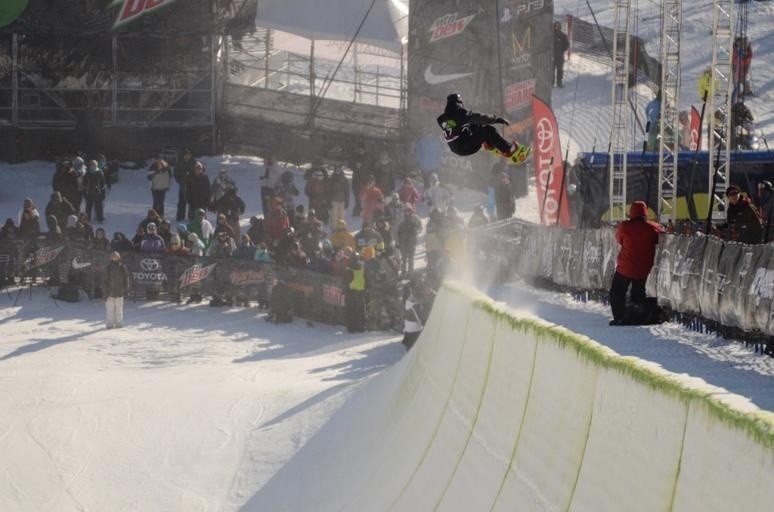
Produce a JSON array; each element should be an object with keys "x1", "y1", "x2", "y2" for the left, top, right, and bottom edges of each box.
[{"x1": 480, "y1": 138, "x2": 532, "y2": 165}]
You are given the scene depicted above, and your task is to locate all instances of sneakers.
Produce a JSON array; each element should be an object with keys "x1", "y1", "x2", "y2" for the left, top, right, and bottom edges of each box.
[{"x1": 506, "y1": 141, "x2": 519, "y2": 159}]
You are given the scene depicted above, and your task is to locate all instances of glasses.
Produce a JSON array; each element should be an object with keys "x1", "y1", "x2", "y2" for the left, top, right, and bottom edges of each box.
[{"x1": 139, "y1": 156, "x2": 377, "y2": 267}]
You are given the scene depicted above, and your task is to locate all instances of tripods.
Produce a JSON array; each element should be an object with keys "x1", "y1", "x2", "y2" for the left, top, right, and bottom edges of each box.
[{"x1": 11, "y1": 253, "x2": 60, "y2": 312}]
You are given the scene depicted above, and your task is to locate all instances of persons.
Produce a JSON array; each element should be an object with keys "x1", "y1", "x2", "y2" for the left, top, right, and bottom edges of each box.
[
  {"x1": 732, "y1": 37, "x2": 754, "y2": 97},
  {"x1": 607, "y1": 200, "x2": 665, "y2": 327},
  {"x1": 758, "y1": 180, "x2": 774, "y2": 243},
  {"x1": 551, "y1": 21, "x2": 570, "y2": 89},
  {"x1": 467, "y1": 203, "x2": 489, "y2": 227},
  {"x1": 644, "y1": 89, "x2": 662, "y2": 153},
  {"x1": 436, "y1": 93, "x2": 531, "y2": 164},
  {"x1": 494, "y1": 171, "x2": 516, "y2": 221},
  {"x1": 715, "y1": 185, "x2": 762, "y2": 244},
  {"x1": 700, "y1": 66, "x2": 720, "y2": 103}
]
